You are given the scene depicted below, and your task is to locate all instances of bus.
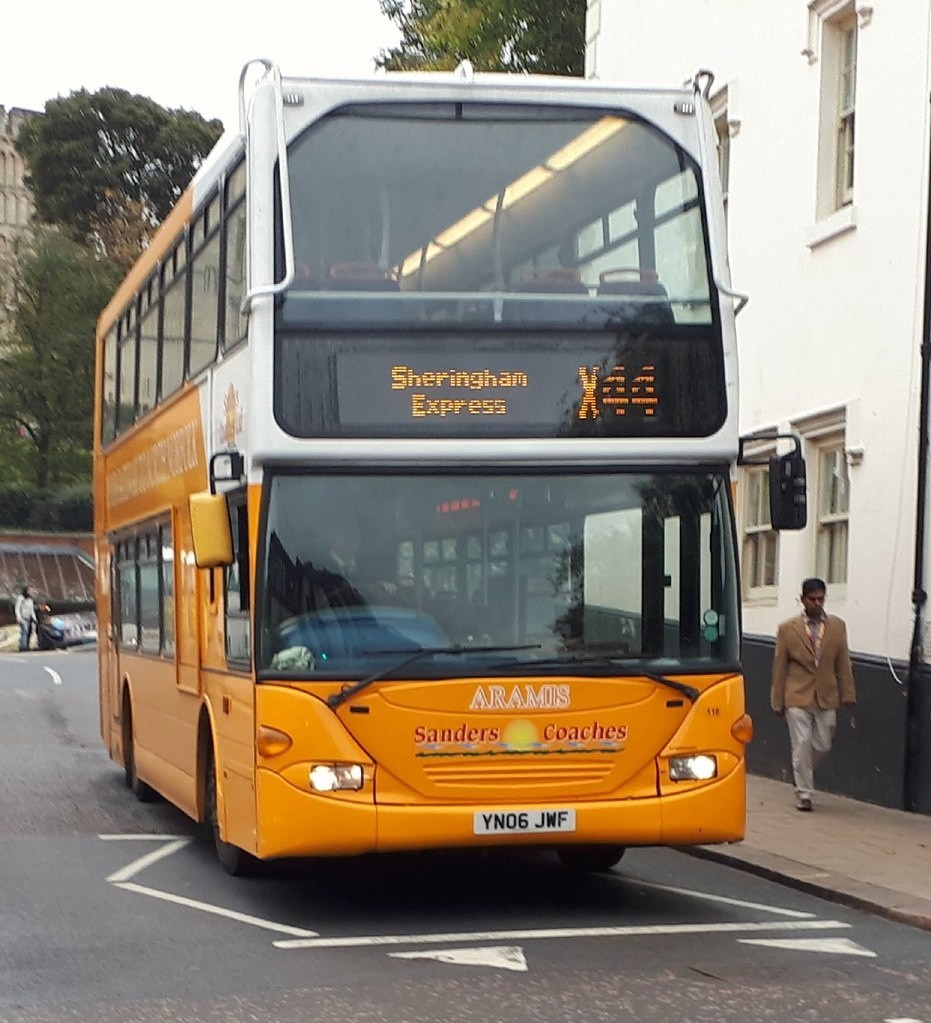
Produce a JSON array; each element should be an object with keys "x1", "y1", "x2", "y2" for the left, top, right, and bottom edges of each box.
[{"x1": 83, "y1": 56, "x2": 809, "y2": 885}]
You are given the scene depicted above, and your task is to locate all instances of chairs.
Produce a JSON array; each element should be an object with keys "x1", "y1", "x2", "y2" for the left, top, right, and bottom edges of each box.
[
  {"x1": 269, "y1": 570, "x2": 500, "y2": 632},
  {"x1": 285, "y1": 253, "x2": 679, "y2": 329}
]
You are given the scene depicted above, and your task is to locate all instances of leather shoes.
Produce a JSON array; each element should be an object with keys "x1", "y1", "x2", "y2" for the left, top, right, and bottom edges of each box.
[{"x1": 796, "y1": 798, "x2": 812, "y2": 810}]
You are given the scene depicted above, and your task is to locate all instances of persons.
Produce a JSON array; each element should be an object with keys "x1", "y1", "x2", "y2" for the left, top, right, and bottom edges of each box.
[
  {"x1": 770, "y1": 576, "x2": 856, "y2": 810},
  {"x1": 299, "y1": 506, "x2": 400, "y2": 610},
  {"x1": 13, "y1": 584, "x2": 39, "y2": 651}
]
